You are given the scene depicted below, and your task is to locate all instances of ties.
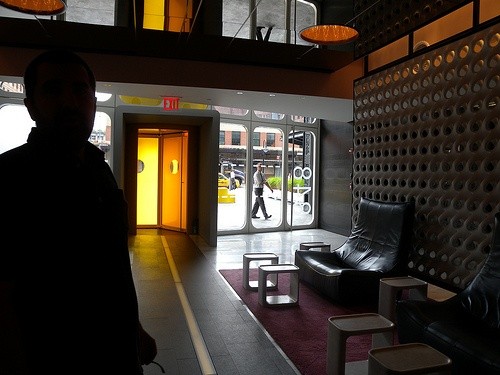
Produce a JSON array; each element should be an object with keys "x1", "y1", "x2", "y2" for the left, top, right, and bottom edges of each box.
[{"x1": 260, "y1": 173, "x2": 263, "y2": 182}]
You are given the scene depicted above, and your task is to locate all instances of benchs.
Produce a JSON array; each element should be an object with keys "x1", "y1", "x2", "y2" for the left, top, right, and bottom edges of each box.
[
  {"x1": 397, "y1": 213, "x2": 500, "y2": 375},
  {"x1": 295, "y1": 198, "x2": 405, "y2": 307}
]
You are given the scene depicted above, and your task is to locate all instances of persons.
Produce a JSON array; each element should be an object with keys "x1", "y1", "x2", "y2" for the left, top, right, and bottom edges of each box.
[
  {"x1": 252, "y1": 163, "x2": 273, "y2": 220},
  {"x1": 288, "y1": 172, "x2": 292, "y2": 179},
  {"x1": 231, "y1": 171, "x2": 235, "y2": 190},
  {"x1": 0, "y1": 47, "x2": 157, "y2": 375}
]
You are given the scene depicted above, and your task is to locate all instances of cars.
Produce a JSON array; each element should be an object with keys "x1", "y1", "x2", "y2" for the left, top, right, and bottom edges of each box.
[{"x1": 217, "y1": 161, "x2": 245, "y2": 191}]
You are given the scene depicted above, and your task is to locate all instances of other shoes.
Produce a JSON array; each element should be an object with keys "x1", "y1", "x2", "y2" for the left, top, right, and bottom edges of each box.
[
  {"x1": 251, "y1": 217, "x2": 260, "y2": 218},
  {"x1": 266, "y1": 215, "x2": 272, "y2": 219}
]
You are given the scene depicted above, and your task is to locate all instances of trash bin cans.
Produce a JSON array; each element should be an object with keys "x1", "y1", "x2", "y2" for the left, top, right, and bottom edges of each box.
[{"x1": 303, "y1": 190, "x2": 312, "y2": 215}]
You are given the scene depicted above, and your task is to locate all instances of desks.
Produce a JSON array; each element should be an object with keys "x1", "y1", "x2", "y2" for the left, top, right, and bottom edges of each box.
[
  {"x1": 243, "y1": 252, "x2": 279, "y2": 291},
  {"x1": 326, "y1": 312, "x2": 395, "y2": 375},
  {"x1": 366, "y1": 343, "x2": 453, "y2": 375},
  {"x1": 257, "y1": 264, "x2": 300, "y2": 308},
  {"x1": 379, "y1": 277, "x2": 429, "y2": 324},
  {"x1": 301, "y1": 241, "x2": 331, "y2": 252}
]
